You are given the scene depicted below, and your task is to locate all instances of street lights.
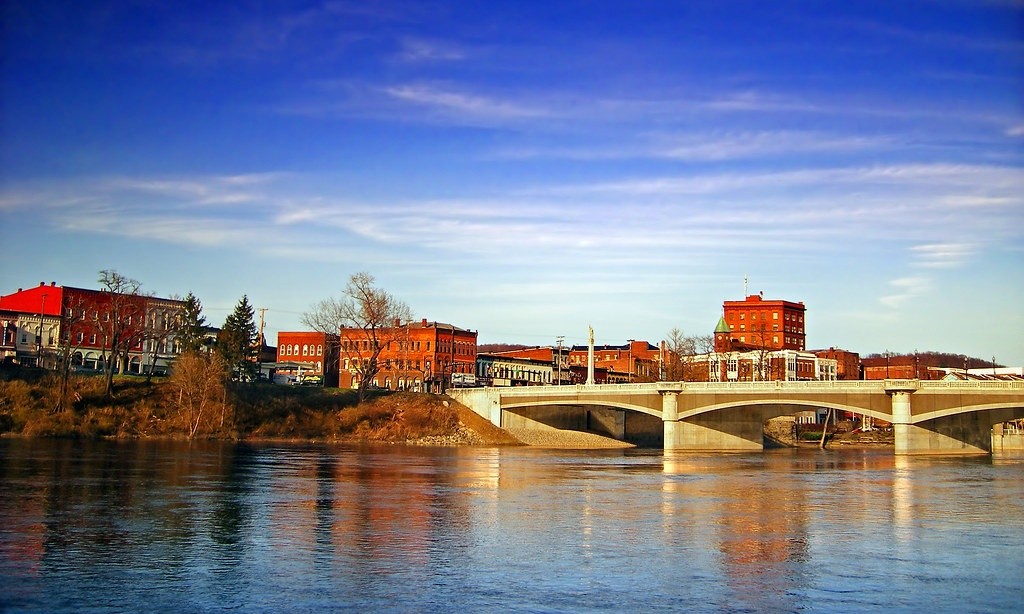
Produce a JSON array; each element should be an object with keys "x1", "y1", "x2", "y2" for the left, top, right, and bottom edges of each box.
[
  {"x1": 555, "y1": 336, "x2": 565, "y2": 385},
  {"x1": 914, "y1": 349, "x2": 918, "y2": 378},
  {"x1": 964, "y1": 356, "x2": 969, "y2": 380},
  {"x1": 777, "y1": 353, "x2": 781, "y2": 380},
  {"x1": 626, "y1": 340, "x2": 635, "y2": 383},
  {"x1": 884, "y1": 350, "x2": 890, "y2": 379},
  {"x1": 992, "y1": 356, "x2": 996, "y2": 380}
]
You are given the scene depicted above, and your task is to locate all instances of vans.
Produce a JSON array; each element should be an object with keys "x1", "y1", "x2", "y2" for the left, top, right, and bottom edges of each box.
[{"x1": 272, "y1": 373, "x2": 297, "y2": 385}]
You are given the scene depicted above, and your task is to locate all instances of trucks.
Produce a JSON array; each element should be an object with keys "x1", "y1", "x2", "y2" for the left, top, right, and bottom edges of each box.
[{"x1": 232, "y1": 374, "x2": 254, "y2": 383}]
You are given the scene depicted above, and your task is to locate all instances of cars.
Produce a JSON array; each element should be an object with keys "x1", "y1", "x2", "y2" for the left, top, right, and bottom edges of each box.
[{"x1": 152, "y1": 370, "x2": 168, "y2": 376}]
